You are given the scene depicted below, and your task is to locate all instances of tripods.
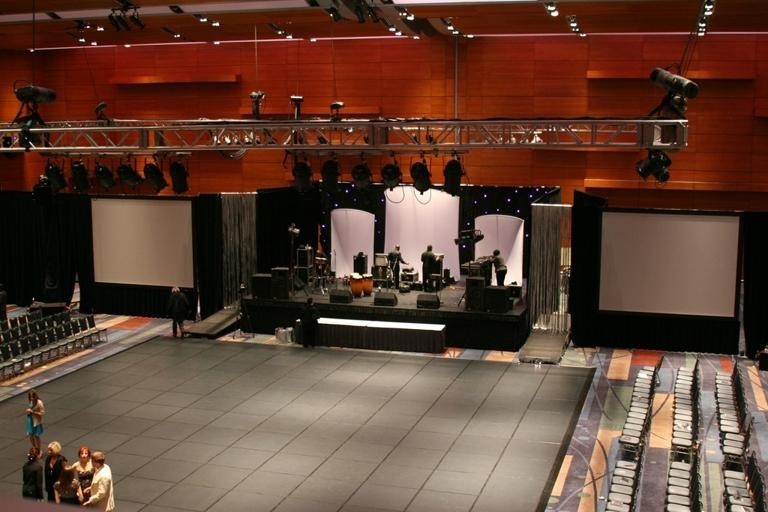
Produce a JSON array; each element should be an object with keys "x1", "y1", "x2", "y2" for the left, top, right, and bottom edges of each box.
[
  {"x1": 458, "y1": 260, "x2": 476, "y2": 311},
  {"x1": 312, "y1": 271, "x2": 328, "y2": 296},
  {"x1": 232, "y1": 296, "x2": 254, "y2": 339}
]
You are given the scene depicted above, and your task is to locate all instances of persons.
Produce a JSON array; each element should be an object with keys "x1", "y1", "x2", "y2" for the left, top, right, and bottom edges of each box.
[
  {"x1": 23, "y1": 441, "x2": 115, "y2": 511},
  {"x1": 387, "y1": 244, "x2": 409, "y2": 289},
  {"x1": 26, "y1": 390, "x2": 45, "y2": 460},
  {"x1": 169, "y1": 286, "x2": 185, "y2": 339},
  {"x1": 302, "y1": 297, "x2": 321, "y2": 347},
  {"x1": 420, "y1": 245, "x2": 436, "y2": 292},
  {"x1": 489, "y1": 249, "x2": 507, "y2": 286}
]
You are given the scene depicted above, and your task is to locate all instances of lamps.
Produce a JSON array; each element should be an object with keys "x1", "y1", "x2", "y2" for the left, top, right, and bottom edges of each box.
[
  {"x1": 108, "y1": 7, "x2": 145, "y2": 31},
  {"x1": 209, "y1": 130, "x2": 260, "y2": 146},
  {"x1": 329, "y1": 0, "x2": 379, "y2": 24},
  {"x1": 16, "y1": 86, "x2": 56, "y2": 126},
  {"x1": 646, "y1": 68, "x2": 699, "y2": 120},
  {"x1": 636, "y1": 149, "x2": 672, "y2": 182},
  {"x1": 39, "y1": 152, "x2": 192, "y2": 194},
  {"x1": 282, "y1": 149, "x2": 465, "y2": 196}
]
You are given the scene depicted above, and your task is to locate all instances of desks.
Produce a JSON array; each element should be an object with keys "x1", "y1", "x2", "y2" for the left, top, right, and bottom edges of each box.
[{"x1": 295, "y1": 318, "x2": 448, "y2": 354}]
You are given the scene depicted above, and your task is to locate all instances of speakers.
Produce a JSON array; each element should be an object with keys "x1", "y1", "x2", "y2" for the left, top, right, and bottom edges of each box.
[
  {"x1": 330, "y1": 289, "x2": 353, "y2": 304},
  {"x1": 250, "y1": 273, "x2": 273, "y2": 299},
  {"x1": 485, "y1": 286, "x2": 508, "y2": 314},
  {"x1": 373, "y1": 292, "x2": 398, "y2": 307},
  {"x1": 272, "y1": 267, "x2": 293, "y2": 300},
  {"x1": 465, "y1": 276, "x2": 484, "y2": 313},
  {"x1": 417, "y1": 294, "x2": 441, "y2": 309}
]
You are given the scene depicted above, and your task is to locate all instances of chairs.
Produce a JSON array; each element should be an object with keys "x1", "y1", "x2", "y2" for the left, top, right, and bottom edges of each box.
[
  {"x1": 0, "y1": 309, "x2": 108, "y2": 383},
  {"x1": 604, "y1": 353, "x2": 768, "y2": 512}
]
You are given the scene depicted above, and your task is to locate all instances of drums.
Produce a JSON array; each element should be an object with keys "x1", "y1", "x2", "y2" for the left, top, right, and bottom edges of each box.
[
  {"x1": 352, "y1": 276, "x2": 362, "y2": 298},
  {"x1": 362, "y1": 274, "x2": 373, "y2": 297},
  {"x1": 350, "y1": 273, "x2": 359, "y2": 289}
]
